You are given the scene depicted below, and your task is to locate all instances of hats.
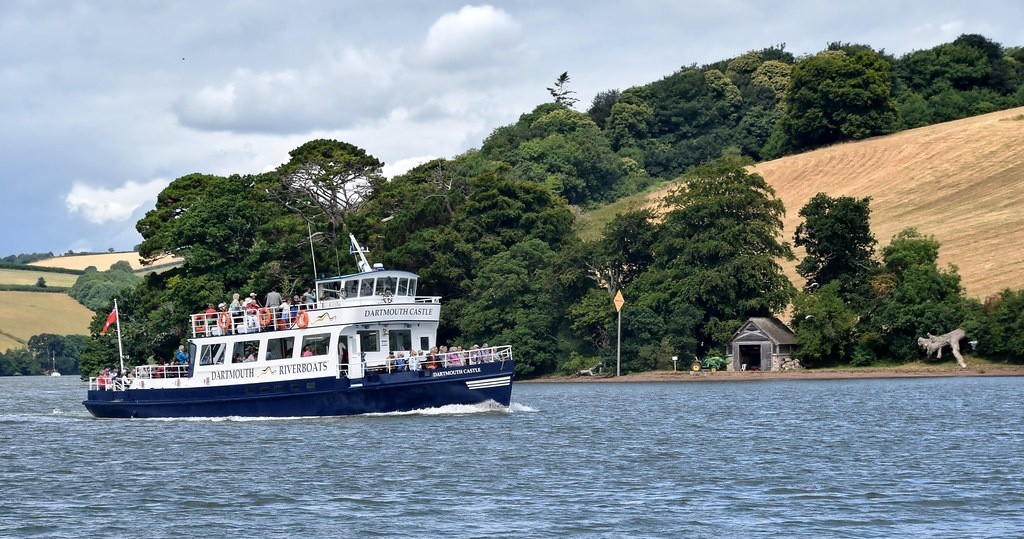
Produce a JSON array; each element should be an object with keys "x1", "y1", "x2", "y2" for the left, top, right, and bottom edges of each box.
[
  {"x1": 250, "y1": 293, "x2": 257, "y2": 297},
  {"x1": 418, "y1": 350, "x2": 423, "y2": 355},
  {"x1": 473, "y1": 345, "x2": 479, "y2": 348}
]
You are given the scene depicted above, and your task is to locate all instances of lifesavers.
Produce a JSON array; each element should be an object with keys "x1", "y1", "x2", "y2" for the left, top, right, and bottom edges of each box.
[
  {"x1": 296, "y1": 310, "x2": 309, "y2": 328},
  {"x1": 195, "y1": 315, "x2": 206, "y2": 331},
  {"x1": 259, "y1": 307, "x2": 271, "y2": 325},
  {"x1": 219, "y1": 313, "x2": 231, "y2": 329}
]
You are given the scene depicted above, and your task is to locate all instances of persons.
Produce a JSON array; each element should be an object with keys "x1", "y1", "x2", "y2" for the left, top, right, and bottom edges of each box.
[
  {"x1": 386, "y1": 345, "x2": 501, "y2": 372},
  {"x1": 205, "y1": 287, "x2": 316, "y2": 333},
  {"x1": 304, "y1": 347, "x2": 313, "y2": 356},
  {"x1": 138, "y1": 362, "x2": 173, "y2": 378},
  {"x1": 242, "y1": 343, "x2": 274, "y2": 361},
  {"x1": 173, "y1": 346, "x2": 189, "y2": 377},
  {"x1": 98, "y1": 368, "x2": 138, "y2": 391},
  {"x1": 338, "y1": 338, "x2": 347, "y2": 376}
]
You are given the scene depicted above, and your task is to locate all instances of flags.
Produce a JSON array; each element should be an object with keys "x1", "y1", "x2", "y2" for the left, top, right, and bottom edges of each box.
[{"x1": 103, "y1": 308, "x2": 117, "y2": 333}]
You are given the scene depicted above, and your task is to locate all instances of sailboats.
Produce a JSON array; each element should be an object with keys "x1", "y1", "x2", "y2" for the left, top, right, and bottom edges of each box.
[{"x1": 39, "y1": 348, "x2": 61, "y2": 376}]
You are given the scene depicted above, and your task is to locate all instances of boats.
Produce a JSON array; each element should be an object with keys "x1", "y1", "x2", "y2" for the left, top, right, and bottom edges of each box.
[{"x1": 81, "y1": 221, "x2": 516, "y2": 421}]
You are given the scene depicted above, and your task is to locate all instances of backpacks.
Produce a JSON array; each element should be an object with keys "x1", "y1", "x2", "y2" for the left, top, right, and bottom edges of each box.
[{"x1": 173, "y1": 353, "x2": 183, "y2": 364}]
